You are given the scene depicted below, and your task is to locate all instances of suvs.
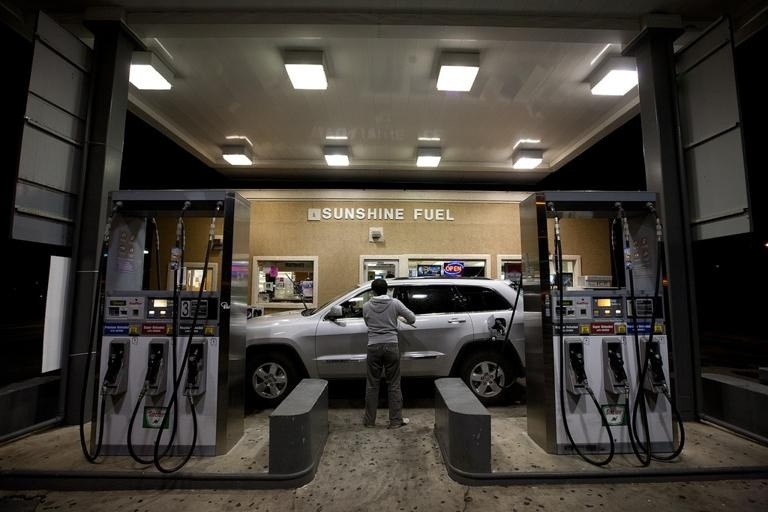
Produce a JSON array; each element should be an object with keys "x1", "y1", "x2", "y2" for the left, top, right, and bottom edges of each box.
[{"x1": 245, "y1": 273, "x2": 529, "y2": 409}]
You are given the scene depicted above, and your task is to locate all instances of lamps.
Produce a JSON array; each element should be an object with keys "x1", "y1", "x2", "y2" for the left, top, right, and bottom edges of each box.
[
  {"x1": 511, "y1": 149, "x2": 543, "y2": 169},
  {"x1": 284, "y1": 50, "x2": 329, "y2": 92},
  {"x1": 416, "y1": 148, "x2": 442, "y2": 167},
  {"x1": 589, "y1": 56, "x2": 639, "y2": 97},
  {"x1": 431, "y1": 51, "x2": 480, "y2": 92},
  {"x1": 222, "y1": 146, "x2": 253, "y2": 166},
  {"x1": 128, "y1": 51, "x2": 175, "y2": 91},
  {"x1": 323, "y1": 146, "x2": 349, "y2": 167}
]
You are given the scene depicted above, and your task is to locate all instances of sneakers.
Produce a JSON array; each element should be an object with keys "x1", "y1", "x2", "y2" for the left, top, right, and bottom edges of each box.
[
  {"x1": 391, "y1": 417, "x2": 409, "y2": 429},
  {"x1": 367, "y1": 425, "x2": 375, "y2": 428}
]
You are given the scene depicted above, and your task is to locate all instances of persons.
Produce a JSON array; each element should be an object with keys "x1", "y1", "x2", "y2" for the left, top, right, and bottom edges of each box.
[{"x1": 362, "y1": 278, "x2": 416, "y2": 429}]
